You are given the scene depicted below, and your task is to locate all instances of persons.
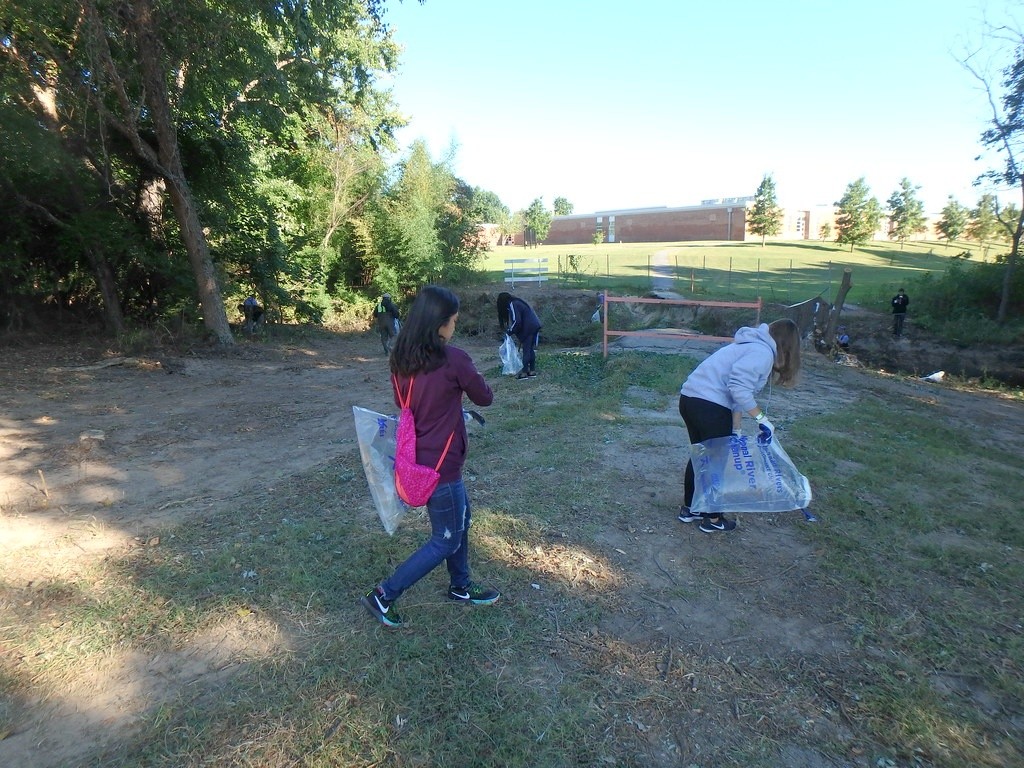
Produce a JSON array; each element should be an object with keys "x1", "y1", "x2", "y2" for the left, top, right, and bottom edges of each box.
[
  {"x1": 588, "y1": 292, "x2": 604, "y2": 323},
  {"x1": 236, "y1": 304, "x2": 265, "y2": 332},
  {"x1": 372, "y1": 293, "x2": 400, "y2": 355},
  {"x1": 360, "y1": 285, "x2": 502, "y2": 627},
  {"x1": 835, "y1": 325, "x2": 850, "y2": 353},
  {"x1": 891, "y1": 288, "x2": 909, "y2": 339},
  {"x1": 243, "y1": 294, "x2": 257, "y2": 336},
  {"x1": 496, "y1": 291, "x2": 542, "y2": 380},
  {"x1": 677, "y1": 317, "x2": 805, "y2": 533},
  {"x1": 813, "y1": 328, "x2": 831, "y2": 356}
]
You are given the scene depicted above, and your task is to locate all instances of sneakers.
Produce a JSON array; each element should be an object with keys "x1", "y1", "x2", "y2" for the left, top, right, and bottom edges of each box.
[
  {"x1": 700, "y1": 516, "x2": 735, "y2": 533},
  {"x1": 359, "y1": 587, "x2": 404, "y2": 628},
  {"x1": 448, "y1": 582, "x2": 499, "y2": 604},
  {"x1": 678, "y1": 505, "x2": 707, "y2": 522}
]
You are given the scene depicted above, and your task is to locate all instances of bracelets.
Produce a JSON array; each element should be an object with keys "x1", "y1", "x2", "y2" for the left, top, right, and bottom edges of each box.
[{"x1": 754, "y1": 412, "x2": 765, "y2": 420}]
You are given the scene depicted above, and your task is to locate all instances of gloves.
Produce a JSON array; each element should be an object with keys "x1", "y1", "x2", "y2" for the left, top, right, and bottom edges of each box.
[
  {"x1": 757, "y1": 414, "x2": 775, "y2": 443},
  {"x1": 462, "y1": 408, "x2": 472, "y2": 421},
  {"x1": 502, "y1": 332, "x2": 507, "y2": 340}
]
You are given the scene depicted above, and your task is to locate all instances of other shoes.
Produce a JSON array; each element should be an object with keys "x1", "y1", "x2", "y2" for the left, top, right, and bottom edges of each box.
[
  {"x1": 529, "y1": 371, "x2": 537, "y2": 376},
  {"x1": 515, "y1": 372, "x2": 527, "y2": 379},
  {"x1": 389, "y1": 343, "x2": 393, "y2": 351},
  {"x1": 386, "y1": 353, "x2": 389, "y2": 357}
]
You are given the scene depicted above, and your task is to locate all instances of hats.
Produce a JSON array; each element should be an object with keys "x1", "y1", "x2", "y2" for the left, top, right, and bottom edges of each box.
[
  {"x1": 250, "y1": 294, "x2": 256, "y2": 299},
  {"x1": 383, "y1": 292, "x2": 390, "y2": 297}
]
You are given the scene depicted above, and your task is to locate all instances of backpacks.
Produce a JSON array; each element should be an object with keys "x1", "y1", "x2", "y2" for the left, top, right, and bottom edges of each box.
[{"x1": 392, "y1": 370, "x2": 457, "y2": 507}]
